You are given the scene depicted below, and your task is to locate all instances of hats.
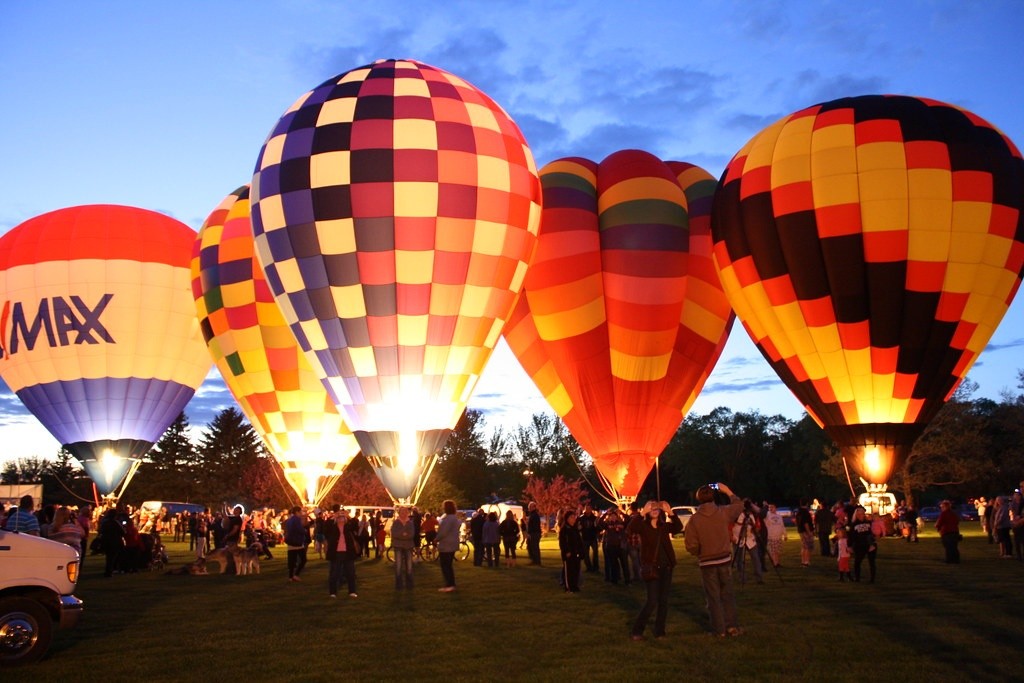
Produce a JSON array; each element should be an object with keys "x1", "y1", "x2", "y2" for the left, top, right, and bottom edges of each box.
[{"x1": 939, "y1": 500, "x2": 950, "y2": 506}]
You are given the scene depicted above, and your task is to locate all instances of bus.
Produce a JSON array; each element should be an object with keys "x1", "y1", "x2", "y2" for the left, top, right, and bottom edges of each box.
[
  {"x1": 139, "y1": 500, "x2": 209, "y2": 533},
  {"x1": 343, "y1": 504, "x2": 400, "y2": 536}
]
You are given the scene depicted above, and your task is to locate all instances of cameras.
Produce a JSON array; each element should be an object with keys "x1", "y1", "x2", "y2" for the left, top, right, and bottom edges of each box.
[
  {"x1": 651, "y1": 503, "x2": 661, "y2": 510},
  {"x1": 708, "y1": 483, "x2": 720, "y2": 490}
]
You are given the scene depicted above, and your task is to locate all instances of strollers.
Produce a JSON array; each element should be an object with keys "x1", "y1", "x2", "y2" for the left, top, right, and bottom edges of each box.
[{"x1": 112, "y1": 531, "x2": 166, "y2": 574}]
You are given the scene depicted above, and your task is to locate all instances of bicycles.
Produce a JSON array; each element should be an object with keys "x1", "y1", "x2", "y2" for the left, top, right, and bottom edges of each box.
[
  {"x1": 385, "y1": 533, "x2": 440, "y2": 564},
  {"x1": 452, "y1": 531, "x2": 501, "y2": 562}
]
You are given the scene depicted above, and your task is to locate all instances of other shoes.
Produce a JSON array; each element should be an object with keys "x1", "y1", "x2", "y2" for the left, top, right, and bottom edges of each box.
[
  {"x1": 350, "y1": 593, "x2": 357, "y2": 597},
  {"x1": 713, "y1": 626, "x2": 738, "y2": 639},
  {"x1": 657, "y1": 635, "x2": 667, "y2": 641},
  {"x1": 331, "y1": 593, "x2": 336, "y2": 597},
  {"x1": 629, "y1": 632, "x2": 645, "y2": 642},
  {"x1": 438, "y1": 585, "x2": 456, "y2": 593},
  {"x1": 801, "y1": 562, "x2": 809, "y2": 568},
  {"x1": 288, "y1": 574, "x2": 301, "y2": 582},
  {"x1": 475, "y1": 560, "x2": 641, "y2": 595}
]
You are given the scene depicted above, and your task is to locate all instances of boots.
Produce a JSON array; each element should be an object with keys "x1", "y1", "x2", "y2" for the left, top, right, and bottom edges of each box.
[
  {"x1": 847, "y1": 572, "x2": 854, "y2": 581},
  {"x1": 869, "y1": 568, "x2": 875, "y2": 584},
  {"x1": 855, "y1": 568, "x2": 861, "y2": 582},
  {"x1": 839, "y1": 572, "x2": 844, "y2": 581}
]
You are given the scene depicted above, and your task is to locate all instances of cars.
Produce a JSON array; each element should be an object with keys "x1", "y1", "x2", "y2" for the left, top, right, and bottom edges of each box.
[
  {"x1": 919, "y1": 506, "x2": 942, "y2": 521},
  {"x1": 774, "y1": 506, "x2": 797, "y2": 527},
  {"x1": 954, "y1": 504, "x2": 979, "y2": 521}
]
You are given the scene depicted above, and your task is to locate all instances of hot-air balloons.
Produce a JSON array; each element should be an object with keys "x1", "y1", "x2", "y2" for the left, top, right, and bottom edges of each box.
[
  {"x1": 0, "y1": 202, "x2": 217, "y2": 517},
  {"x1": 497, "y1": 146, "x2": 737, "y2": 516},
  {"x1": 190, "y1": 182, "x2": 361, "y2": 521},
  {"x1": 707, "y1": 90, "x2": 1023, "y2": 538},
  {"x1": 244, "y1": 54, "x2": 545, "y2": 518}
]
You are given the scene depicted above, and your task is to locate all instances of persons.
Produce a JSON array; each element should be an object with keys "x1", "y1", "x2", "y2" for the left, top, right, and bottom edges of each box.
[
  {"x1": 140, "y1": 506, "x2": 171, "y2": 535},
  {"x1": 729, "y1": 498, "x2": 788, "y2": 583},
  {"x1": 281, "y1": 506, "x2": 315, "y2": 581},
  {"x1": 244, "y1": 507, "x2": 274, "y2": 560},
  {"x1": 35, "y1": 503, "x2": 91, "y2": 568},
  {"x1": 556, "y1": 505, "x2": 602, "y2": 595},
  {"x1": 174, "y1": 509, "x2": 190, "y2": 542},
  {"x1": 432, "y1": 500, "x2": 459, "y2": 592},
  {"x1": 974, "y1": 481, "x2": 1024, "y2": 557},
  {"x1": 797, "y1": 496, "x2": 878, "y2": 583},
  {"x1": 934, "y1": 500, "x2": 960, "y2": 563},
  {"x1": 519, "y1": 502, "x2": 541, "y2": 565},
  {"x1": 460, "y1": 508, "x2": 520, "y2": 568},
  {"x1": 208, "y1": 500, "x2": 242, "y2": 574},
  {"x1": 100, "y1": 500, "x2": 145, "y2": 578},
  {"x1": 312, "y1": 503, "x2": 360, "y2": 598},
  {"x1": 684, "y1": 482, "x2": 745, "y2": 636},
  {"x1": 597, "y1": 501, "x2": 683, "y2": 641},
  {"x1": 391, "y1": 506, "x2": 438, "y2": 591},
  {"x1": 0, "y1": 494, "x2": 40, "y2": 537},
  {"x1": 189, "y1": 506, "x2": 213, "y2": 552},
  {"x1": 352, "y1": 510, "x2": 386, "y2": 558},
  {"x1": 865, "y1": 499, "x2": 919, "y2": 544}
]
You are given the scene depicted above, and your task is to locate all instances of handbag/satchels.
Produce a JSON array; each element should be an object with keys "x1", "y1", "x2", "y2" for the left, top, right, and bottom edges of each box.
[
  {"x1": 349, "y1": 531, "x2": 361, "y2": 555},
  {"x1": 992, "y1": 528, "x2": 999, "y2": 544},
  {"x1": 288, "y1": 529, "x2": 304, "y2": 545},
  {"x1": 640, "y1": 561, "x2": 667, "y2": 579},
  {"x1": 1013, "y1": 517, "x2": 1024, "y2": 527}
]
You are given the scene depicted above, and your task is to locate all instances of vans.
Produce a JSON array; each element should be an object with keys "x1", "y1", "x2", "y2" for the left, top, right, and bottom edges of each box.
[{"x1": 856, "y1": 492, "x2": 899, "y2": 519}]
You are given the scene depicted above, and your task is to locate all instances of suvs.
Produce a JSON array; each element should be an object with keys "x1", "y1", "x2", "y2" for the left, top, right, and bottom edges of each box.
[
  {"x1": 0, "y1": 529, "x2": 85, "y2": 667},
  {"x1": 668, "y1": 505, "x2": 700, "y2": 536}
]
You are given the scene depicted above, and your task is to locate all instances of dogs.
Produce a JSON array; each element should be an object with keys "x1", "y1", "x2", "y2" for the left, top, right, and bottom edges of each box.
[{"x1": 223, "y1": 541, "x2": 263, "y2": 576}]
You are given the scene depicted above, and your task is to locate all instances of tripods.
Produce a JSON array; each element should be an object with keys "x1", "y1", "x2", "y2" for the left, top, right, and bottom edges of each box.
[{"x1": 731, "y1": 510, "x2": 784, "y2": 589}]
